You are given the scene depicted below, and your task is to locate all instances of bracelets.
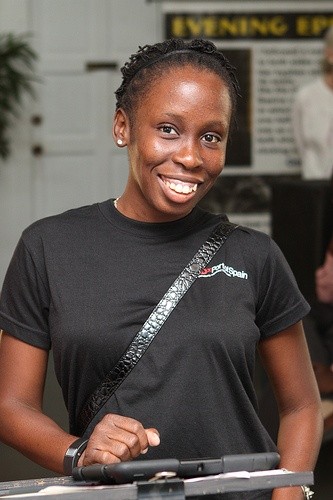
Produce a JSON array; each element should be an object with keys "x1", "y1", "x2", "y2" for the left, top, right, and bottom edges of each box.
[
  {"x1": 63, "y1": 437, "x2": 89, "y2": 476},
  {"x1": 282, "y1": 468, "x2": 315, "y2": 500}
]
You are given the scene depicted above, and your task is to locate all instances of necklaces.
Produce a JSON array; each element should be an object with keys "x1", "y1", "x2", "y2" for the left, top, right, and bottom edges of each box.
[{"x1": 113, "y1": 196, "x2": 121, "y2": 208}]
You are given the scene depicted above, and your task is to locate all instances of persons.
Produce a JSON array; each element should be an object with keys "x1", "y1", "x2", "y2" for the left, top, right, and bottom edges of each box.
[
  {"x1": 0, "y1": 37, "x2": 324, "y2": 500},
  {"x1": 287, "y1": 45, "x2": 332, "y2": 338}
]
